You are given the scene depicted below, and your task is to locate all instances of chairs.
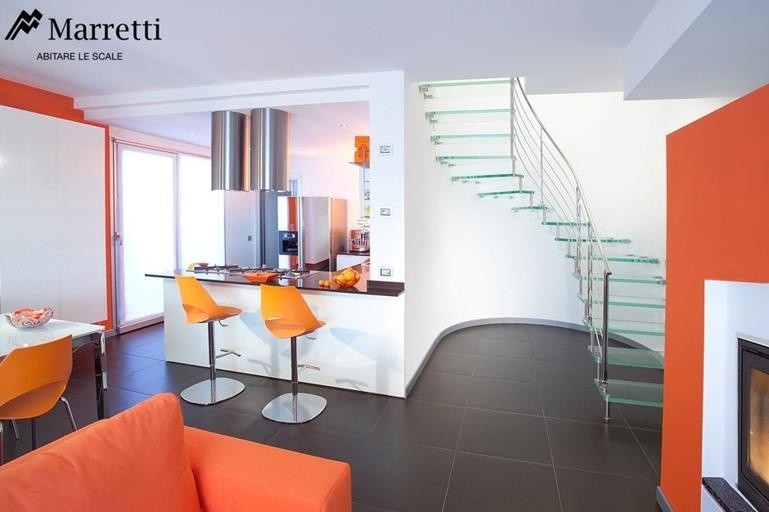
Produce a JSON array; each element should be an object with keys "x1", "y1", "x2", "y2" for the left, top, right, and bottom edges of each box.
[
  {"x1": 0, "y1": 391, "x2": 353, "y2": 511},
  {"x1": 1, "y1": 334, "x2": 77, "y2": 452},
  {"x1": 175, "y1": 274, "x2": 327, "y2": 424}
]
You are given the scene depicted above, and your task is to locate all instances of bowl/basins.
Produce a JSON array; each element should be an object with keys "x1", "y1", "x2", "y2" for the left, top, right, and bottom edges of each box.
[
  {"x1": 4, "y1": 306, "x2": 53, "y2": 330},
  {"x1": 332, "y1": 276, "x2": 360, "y2": 289}
]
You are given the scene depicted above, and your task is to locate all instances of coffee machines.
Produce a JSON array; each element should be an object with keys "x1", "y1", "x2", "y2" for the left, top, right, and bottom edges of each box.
[{"x1": 349, "y1": 229, "x2": 370, "y2": 252}]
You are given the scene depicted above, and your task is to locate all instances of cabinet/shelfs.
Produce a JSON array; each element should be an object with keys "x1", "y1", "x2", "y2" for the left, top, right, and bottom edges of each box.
[
  {"x1": 335, "y1": 254, "x2": 370, "y2": 272},
  {"x1": 361, "y1": 149, "x2": 370, "y2": 231}
]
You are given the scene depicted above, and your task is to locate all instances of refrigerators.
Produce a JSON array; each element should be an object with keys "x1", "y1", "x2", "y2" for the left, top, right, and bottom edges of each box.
[{"x1": 277, "y1": 195, "x2": 348, "y2": 273}]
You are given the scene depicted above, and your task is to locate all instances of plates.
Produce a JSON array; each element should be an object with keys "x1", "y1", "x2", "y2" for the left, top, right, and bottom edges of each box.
[{"x1": 244, "y1": 272, "x2": 279, "y2": 284}]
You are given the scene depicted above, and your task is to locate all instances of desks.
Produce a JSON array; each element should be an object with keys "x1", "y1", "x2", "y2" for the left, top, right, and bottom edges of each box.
[{"x1": 0, "y1": 312, "x2": 106, "y2": 421}]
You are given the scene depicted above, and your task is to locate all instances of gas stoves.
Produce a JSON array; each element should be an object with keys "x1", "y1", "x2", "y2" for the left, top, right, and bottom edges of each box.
[{"x1": 194, "y1": 264, "x2": 272, "y2": 273}]
